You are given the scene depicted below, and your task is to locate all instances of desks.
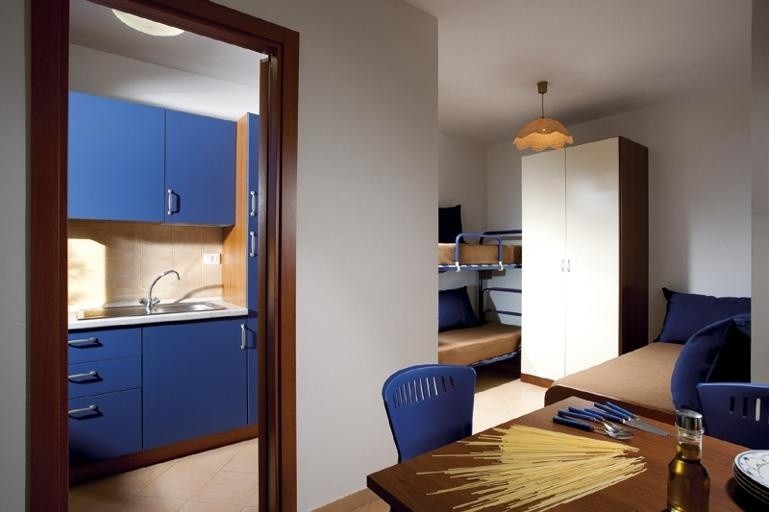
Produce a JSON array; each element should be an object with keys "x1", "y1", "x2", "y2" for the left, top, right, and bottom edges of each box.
[{"x1": 367, "y1": 395, "x2": 759, "y2": 512}]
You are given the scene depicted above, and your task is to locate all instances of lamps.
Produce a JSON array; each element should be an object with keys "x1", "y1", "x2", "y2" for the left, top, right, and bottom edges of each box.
[{"x1": 513, "y1": 80, "x2": 574, "y2": 151}]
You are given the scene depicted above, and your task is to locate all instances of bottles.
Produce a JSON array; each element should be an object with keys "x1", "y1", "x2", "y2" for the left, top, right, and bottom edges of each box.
[{"x1": 663, "y1": 407, "x2": 713, "y2": 512}]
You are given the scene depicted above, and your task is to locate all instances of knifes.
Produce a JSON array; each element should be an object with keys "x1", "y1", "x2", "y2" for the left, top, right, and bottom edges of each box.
[{"x1": 582, "y1": 397, "x2": 670, "y2": 438}]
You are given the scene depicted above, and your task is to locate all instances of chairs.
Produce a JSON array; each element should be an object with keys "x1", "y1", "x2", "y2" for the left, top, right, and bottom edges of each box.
[
  {"x1": 382, "y1": 364, "x2": 476, "y2": 463},
  {"x1": 696, "y1": 383, "x2": 769, "y2": 450}
]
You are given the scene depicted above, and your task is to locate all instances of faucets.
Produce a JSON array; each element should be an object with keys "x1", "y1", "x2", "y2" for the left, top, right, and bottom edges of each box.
[{"x1": 138, "y1": 268, "x2": 182, "y2": 314}]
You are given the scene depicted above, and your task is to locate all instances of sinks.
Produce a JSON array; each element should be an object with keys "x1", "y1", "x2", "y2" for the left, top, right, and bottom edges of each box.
[{"x1": 72, "y1": 299, "x2": 230, "y2": 321}]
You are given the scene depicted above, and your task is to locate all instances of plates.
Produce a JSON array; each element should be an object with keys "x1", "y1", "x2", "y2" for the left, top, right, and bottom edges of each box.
[{"x1": 730, "y1": 447, "x2": 768, "y2": 506}]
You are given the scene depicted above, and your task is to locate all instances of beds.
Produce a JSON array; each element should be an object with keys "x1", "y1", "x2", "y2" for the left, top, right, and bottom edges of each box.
[
  {"x1": 544, "y1": 342, "x2": 686, "y2": 426},
  {"x1": 438, "y1": 230, "x2": 522, "y2": 366}
]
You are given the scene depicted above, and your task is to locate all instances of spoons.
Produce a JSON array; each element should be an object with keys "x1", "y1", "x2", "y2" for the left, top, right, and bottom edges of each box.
[{"x1": 551, "y1": 406, "x2": 635, "y2": 442}]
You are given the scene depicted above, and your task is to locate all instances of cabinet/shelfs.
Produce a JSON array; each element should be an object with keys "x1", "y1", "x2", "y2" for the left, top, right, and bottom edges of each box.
[
  {"x1": 521, "y1": 137, "x2": 649, "y2": 388},
  {"x1": 67, "y1": 327, "x2": 142, "y2": 463},
  {"x1": 67, "y1": 91, "x2": 237, "y2": 228},
  {"x1": 221, "y1": 112, "x2": 259, "y2": 427},
  {"x1": 142, "y1": 318, "x2": 248, "y2": 449}
]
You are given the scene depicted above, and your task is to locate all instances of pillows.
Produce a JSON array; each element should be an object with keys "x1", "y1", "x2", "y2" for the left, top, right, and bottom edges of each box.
[
  {"x1": 439, "y1": 285, "x2": 478, "y2": 330},
  {"x1": 438, "y1": 204, "x2": 467, "y2": 244},
  {"x1": 653, "y1": 287, "x2": 751, "y2": 345},
  {"x1": 670, "y1": 313, "x2": 751, "y2": 412}
]
